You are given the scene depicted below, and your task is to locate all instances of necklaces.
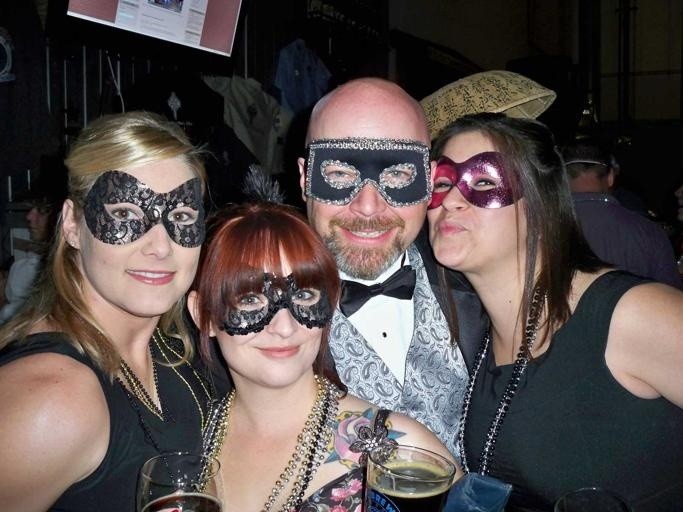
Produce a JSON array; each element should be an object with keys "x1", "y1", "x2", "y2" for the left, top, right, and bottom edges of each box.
[
  {"x1": 197, "y1": 371, "x2": 340, "y2": 512},
  {"x1": 107, "y1": 316, "x2": 210, "y2": 460},
  {"x1": 457, "y1": 277, "x2": 547, "y2": 480}
]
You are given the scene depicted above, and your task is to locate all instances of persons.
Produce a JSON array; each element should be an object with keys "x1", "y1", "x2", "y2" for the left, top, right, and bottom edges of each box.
[
  {"x1": 183, "y1": 196, "x2": 462, "y2": 511},
  {"x1": 0, "y1": 187, "x2": 58, "y2": 325},
  {"x1": 559, "y1": 135, "x2": 679, "y2": 289},
  {"x1": 427, "y1": 113, "x2": 682, "y2": 512},
  {"x1": 287, "y1": 79, "x2": 489, "y2": 470},
  {"x1": 0, "y1": 108, "x2": 230, "y2": 512}
]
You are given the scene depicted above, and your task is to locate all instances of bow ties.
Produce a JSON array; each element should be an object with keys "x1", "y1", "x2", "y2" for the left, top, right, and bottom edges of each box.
[{"x1": 337, "y1": 264, "x2": 418, "y2": 318}]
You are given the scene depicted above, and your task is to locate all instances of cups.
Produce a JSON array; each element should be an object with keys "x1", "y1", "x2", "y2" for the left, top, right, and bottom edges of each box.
[
  {"x1": 135, "y1": 452, "x2": 227, "y2": 512},
  {"x1": 364, "y1": 445, "x2": 456, "y2": 512}
]
